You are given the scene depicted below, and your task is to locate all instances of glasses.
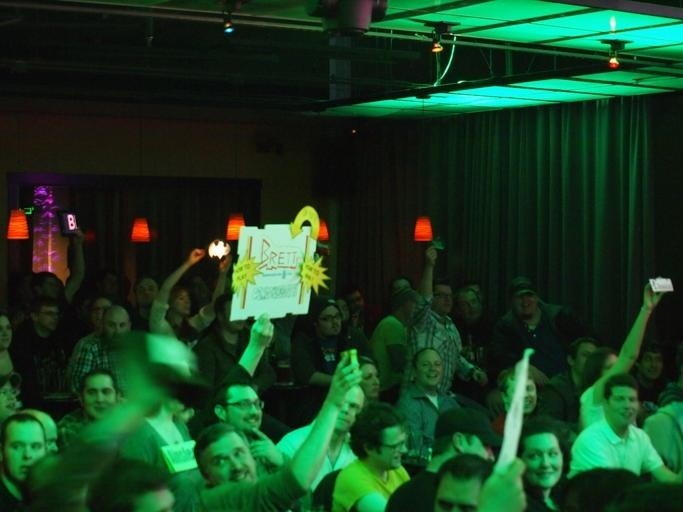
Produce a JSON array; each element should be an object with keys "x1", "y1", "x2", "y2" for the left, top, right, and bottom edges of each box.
[
  {"x1": 37, "y1": 309, "x2": 60, "y2": 317},
  {"x1": 433, "y1": 291, "x2": 458, "y2": 299},
  {"x1": 220, "y1": 400, "x2": 265, "y2": 410},
  {"x1": 376, "y1": 438, "x2": 407, "y2": 450},
  {"x1": 323, "y1": 311, "x2": 341, "y2": 322}
]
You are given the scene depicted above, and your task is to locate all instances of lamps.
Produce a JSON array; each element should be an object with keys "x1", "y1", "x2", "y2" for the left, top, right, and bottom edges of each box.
[
  {"x1": 224, "y1": 134, "x2": 248, "y2": 243},
  {"x1": 316, "y1": 218, "x2": 331, "y2": 241},
  {"x1": 3, "y1": 107, "x2": 30, "y2": 243},
  {"x1": 599, "y1": 38, "x2": 634, "y2": 71},
  {"x1": 222, "y1": 6, "x2": 235, "y2": 35},
  {"x1": 129, "y1": 95, "x2": 151, "y2": 244},
  {"x1": 424, "y1": 21, "x2": 453, "y2": 53},
  {"x1": 413, "y1": 92, "x2": 434, "y2": 243}
]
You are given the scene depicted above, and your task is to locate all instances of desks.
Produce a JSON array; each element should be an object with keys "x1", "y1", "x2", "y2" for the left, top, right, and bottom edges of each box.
[
  {"x1": 452, "y1": 359, "x2": 505, "y2": 406},
  {"x1": 262, "y1": 379, "x2": 318, "y2": 432}
]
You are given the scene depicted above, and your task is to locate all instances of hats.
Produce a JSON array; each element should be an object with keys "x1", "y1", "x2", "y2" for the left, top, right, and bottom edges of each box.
[
  {"x1": 508, "y1": 276, "x2": 537, "y2": 296},
  {"x1": 312, "y1": 295, "x2": 343, "y2": 320},
  {"x1": 432, "y1": 406, "x2": 503, "y2": 450}
]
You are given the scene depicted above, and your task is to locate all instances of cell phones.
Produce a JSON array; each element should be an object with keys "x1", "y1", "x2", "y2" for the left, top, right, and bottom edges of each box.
[
  {"x1": 649, "y1": 278, "x2": 674, "y2": 292},
  {"x1": 340, "y1": 349, "x2": 359, "y2": 373}
]
